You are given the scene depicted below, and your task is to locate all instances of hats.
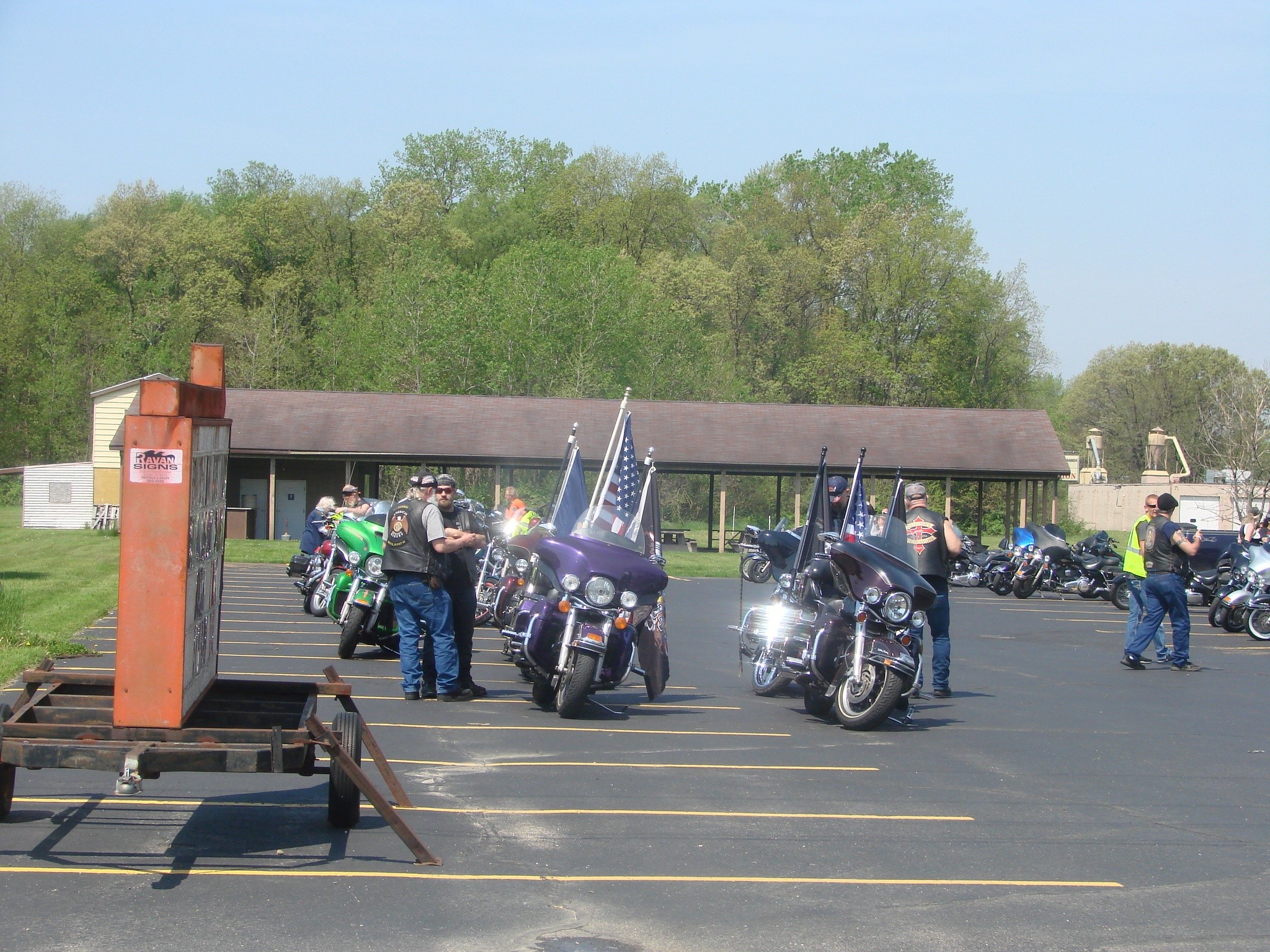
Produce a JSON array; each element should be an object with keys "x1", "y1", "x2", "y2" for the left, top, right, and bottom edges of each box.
[
  {"x1": 904, "y1": 484, "x2": 927, "y2": 500},
  {"x1": 343, "y1": 485, "x2": 358, "y2": 494},
  {"x1": 436, "y1": 474, "x2": 457, "y2": 487},
  {"x1": 828, "y1": 476, "x2": 847, "y2": 503},
  {"x1": 410, "y1": 472, "x2": 436, "y2": 487},
  {"x1": 1248, "y1": 507, "x2": 1260, "y2": 516},
  {"x1": 1157, "y1": 492, "x2": 1178, "y2": 510}
]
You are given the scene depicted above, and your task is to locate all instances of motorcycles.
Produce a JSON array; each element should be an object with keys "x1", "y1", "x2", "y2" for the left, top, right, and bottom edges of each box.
[
  {"x1": 1184, "y1": 534, "x2": 1270, "y2": 642},
  {"x1": 284, "y1": 460, "x2": 549, "y2": 661},
  {"x1": 801, "y1": 513, "x2": 945, "y2": 733},
  {"x1": 946, "y1": 520, "x2": 1131, "y2": 610},
  {"x1": 490, "y1": 504, "x2": 669, "y2": 721},
  {"x1": 728, "y1": 522, "x2": 804, "y2": 583},
  {"x1": 726, "y1": 528, "x2": 832, "y2": 697}
]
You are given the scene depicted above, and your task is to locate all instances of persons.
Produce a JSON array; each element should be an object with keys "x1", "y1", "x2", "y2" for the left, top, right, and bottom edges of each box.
[
  {"x1": 1123, "y1": 494, "x2": 1174, "y2": 663},
  {"x1": 905, "y1": 482, "x2": 963, "y2": 697},
  {"x1": 1120, "y1": 492, "x2": 1201, "y2": 671},
  {"x1": 421, "y1": 473, "x2": 488, "y2": 697},
  {"x1": 871, "y1": 506, "x2": 889, "y2": 537},
  {"x1": 504, "y1": 487, "x2": 526, "y2": 521},
  {"x1": 828, "y1": 476, "x2": 875, "y2": 531},
  {"x1": 381, "y1": 471, "x2": 474, "y2": 702},
  {"x1": 300, "y1": 496, "x2": 338, "y2": 555},
  {"x1": 334, "y1": 484, "x2": 375, "y2": 518},
  {"x1": 1238, "y1": 507, "x2": 1270, "y2": 545}
]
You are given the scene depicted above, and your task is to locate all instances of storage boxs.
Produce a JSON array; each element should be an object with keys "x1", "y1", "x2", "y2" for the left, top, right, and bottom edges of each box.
[{"x1": 286, "y1": 555, "x2": 309, "y2": 578}]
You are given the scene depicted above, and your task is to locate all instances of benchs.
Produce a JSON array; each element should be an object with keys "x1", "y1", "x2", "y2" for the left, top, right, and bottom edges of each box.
[
  {"x1": 687, "y1": 539, "x2": 697, "y2": 551},
  {"x1": 728, "y1": 541, "x2": 740, "y2": 552}
]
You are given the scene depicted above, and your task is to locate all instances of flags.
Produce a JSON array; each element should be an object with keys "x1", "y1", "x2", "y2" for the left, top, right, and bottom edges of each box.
[
  {"x1": 590, "y1": 410, "x2": 640, "y2": 536},
  {"x1": 793, "y1": 457, "x2": 908, "y2": 573},
  {"x1": 626, "y1": 466, "x2": 671, "y2": 701},
  {"x1": 551, "y1": 446, "x2": 590, "y2": 537}
]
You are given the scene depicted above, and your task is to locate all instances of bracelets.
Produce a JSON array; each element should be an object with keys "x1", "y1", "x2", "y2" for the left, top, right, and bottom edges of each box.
[
  {"x1": 344, "y1": 507, "x2": 348, "y2": 513},
  {"x1": 878, "y1": 526, "x2": 883, "y2": 529},
  {"x1": 1196, "y1": 536, "x2": 1201, "y2": 541}
]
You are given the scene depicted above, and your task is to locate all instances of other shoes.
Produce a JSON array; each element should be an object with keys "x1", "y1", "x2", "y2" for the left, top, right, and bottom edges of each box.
[
  {"x1": 1157, "y1": 654, "x2": 1174, "y2": 663},
  {"x1": 437, "y1": 688, "x2": 474, "y2": 701},
  {"x1": 1139, "y1": 656, "x2": 1152, "y2": 664},
  {"x1": 1170, "y1": 661, "x2": 1203, "y2": 671},
  {"x1": 1120, "y1": 654, "x2": 1146, "y2": 670},
  {"x1": 422, "y1": 690, "x2": 437, "y2": 699},
  {"x1": 933, "y1": 687, "x2": 952, "y2": 696},
  {"x1": 461, "y1": 675, "x2": 487, "y2": 695},
  {"x1": 909, "y1": 692, "x2": 919, "y2": 698},
  {"x1": 404, "y1": 691, "x2": 420, "y2": 700}
]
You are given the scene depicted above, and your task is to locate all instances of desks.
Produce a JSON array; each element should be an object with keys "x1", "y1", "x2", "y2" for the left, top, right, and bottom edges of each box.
[
  {"x1": 661, "y1": 529, "x2": 691, "y2": 552},
  {"x1": 739, "y1": 532, "x2": 752, "y2": 544}
]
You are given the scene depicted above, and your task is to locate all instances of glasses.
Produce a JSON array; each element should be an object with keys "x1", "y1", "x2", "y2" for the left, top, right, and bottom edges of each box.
[
  {"x1": 905, "y1": 483, "x2": 924, "y2": 488},
  {"x1": 342, "y1": 491, "x2": 357, "y2": 496},
  {"x1": 436, "y1": 487, "x2": 454, "y2": 494},
  {"x1": 880, "y1": 511, "x2": 888, "y2": 515},
  {"x1": 1146, "y1": 504, "x2": 1156, "y2": 508},
  {"x1": 504, "y1": 494, "x2": 510, "y2": 496}
]
